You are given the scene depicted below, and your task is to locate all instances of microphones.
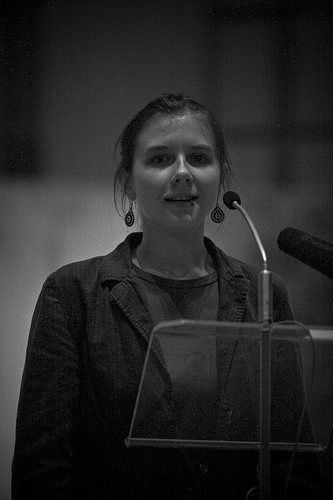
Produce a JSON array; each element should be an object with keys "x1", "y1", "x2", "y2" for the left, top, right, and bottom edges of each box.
[
  {"x1": 277, "y1": 227, "x2": 333, "y2": 278},
  {"x1": 223, "y1": 192, "x2": 274, "y2": 322}
]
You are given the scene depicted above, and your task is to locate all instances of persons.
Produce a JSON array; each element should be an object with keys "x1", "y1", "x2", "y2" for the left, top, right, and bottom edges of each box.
[{"x1": 8, "y1": 91, "x2": 331, "y2": 497}]
